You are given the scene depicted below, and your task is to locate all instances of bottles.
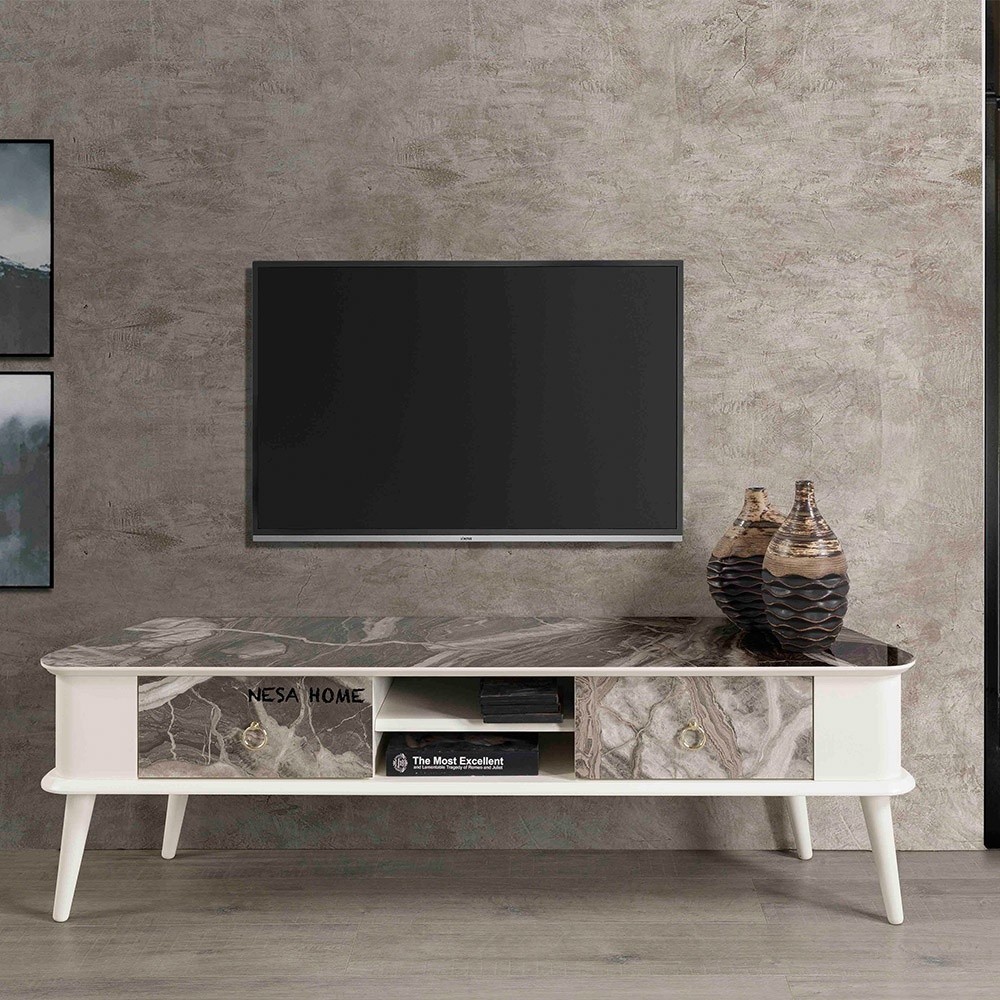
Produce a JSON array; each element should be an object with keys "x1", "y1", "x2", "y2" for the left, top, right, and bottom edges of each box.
[
  {"x1": 762, "y1": 481, "x2": 850, "y2": 653},
  {"x1": 706, "y1": 487, "x2": 787, "y2": 639}
]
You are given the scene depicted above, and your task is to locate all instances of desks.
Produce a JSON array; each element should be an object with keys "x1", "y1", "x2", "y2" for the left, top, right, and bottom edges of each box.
[{"x1": 36, "y1": 614, "x2": 921, "y2": 922}]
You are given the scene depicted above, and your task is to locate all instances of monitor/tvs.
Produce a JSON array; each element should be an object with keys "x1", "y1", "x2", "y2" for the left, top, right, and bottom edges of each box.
[{"x1": 249, "y1": 259, "x2": 683, "y2": 543}]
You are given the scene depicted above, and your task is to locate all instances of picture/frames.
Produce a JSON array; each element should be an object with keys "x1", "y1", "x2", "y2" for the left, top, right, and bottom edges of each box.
[
  {"x1": 0, "y1": 138, "x2": 56, "y2": 359},
  {"x1": 0, "y1": 371, "x2": 54, "y2": 590}
]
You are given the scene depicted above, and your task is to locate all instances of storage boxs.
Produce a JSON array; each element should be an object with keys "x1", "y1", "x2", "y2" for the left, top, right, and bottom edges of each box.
[{"x1": 385, "y1": 733, "x2": 540, "y2": 776}]
